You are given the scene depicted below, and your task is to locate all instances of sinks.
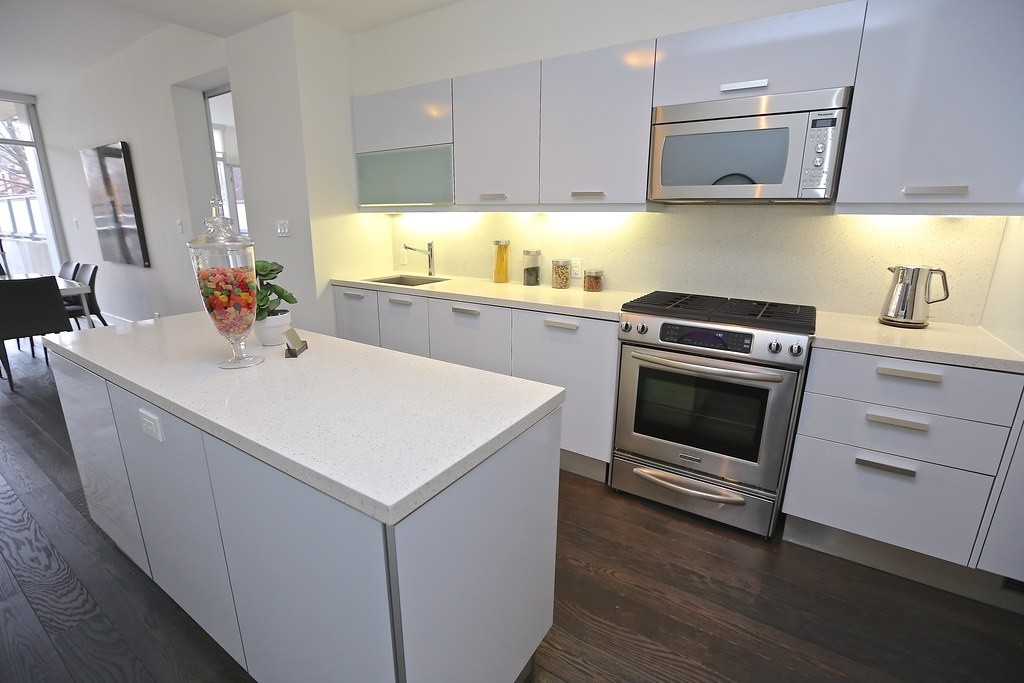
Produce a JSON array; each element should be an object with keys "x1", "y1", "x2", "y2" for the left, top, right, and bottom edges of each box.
[{"x1": 370, "y1": 275, "x2": 451, "y2": 287}]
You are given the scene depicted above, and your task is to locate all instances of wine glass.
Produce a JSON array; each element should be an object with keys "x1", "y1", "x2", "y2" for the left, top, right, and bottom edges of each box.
[{"x1": 186, "y1": 195, "x2": 264, "y2": 369}]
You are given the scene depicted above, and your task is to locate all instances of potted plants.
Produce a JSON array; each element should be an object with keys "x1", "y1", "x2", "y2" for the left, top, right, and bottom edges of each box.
[{"x1": 248, "y1": 260, "x2": 300, "y2": 347}]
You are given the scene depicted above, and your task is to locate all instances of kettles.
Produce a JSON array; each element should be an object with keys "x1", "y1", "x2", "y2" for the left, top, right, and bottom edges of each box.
[{"x1": 877, "y1": 264, "x2": 950, "y2": 329}]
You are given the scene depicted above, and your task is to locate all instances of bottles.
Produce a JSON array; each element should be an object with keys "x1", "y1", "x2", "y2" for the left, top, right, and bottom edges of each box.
[
  {"x1": 492, "y1": 240, "x2": 512, "y2": 282},
  {"x1": 583, "y1": 269, "x2": 602, "y2": 291},
  {"x1": 551, "y1": 259, "x2": 569, "y2": 288},
  {"x1": 523, "y1": 250, "x2": 541, "y2": 286}
]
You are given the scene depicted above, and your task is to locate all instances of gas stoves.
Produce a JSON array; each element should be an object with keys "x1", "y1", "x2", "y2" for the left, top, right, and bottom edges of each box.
[{"x1": 618, "y1": 291, "x2": 816, "y2": 368}]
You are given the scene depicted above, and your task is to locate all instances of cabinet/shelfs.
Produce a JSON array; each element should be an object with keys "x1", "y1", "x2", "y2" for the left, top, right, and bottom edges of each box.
[
  {"x1": 347, "y1": 0, "x2": 1024, "y2": 216},
  {"x1": 331, "y1": 270, "x2": 646, "y2": 488},
  {"x1": 780, "y1": 311, "x2": 1024, "y2": 620}
]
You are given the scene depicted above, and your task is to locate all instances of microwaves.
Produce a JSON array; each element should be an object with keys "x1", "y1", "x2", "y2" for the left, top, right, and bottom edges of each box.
[{"x1": 646, "y1": 86, "x2": 852, "y2": 200}]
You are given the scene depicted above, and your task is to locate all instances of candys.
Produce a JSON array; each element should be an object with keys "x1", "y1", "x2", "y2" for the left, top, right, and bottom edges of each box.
[{"x1": 196, "y1": 266, "x2": 256, "y2": 337}]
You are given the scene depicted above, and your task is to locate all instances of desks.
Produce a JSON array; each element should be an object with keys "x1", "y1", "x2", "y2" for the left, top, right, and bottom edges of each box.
[{"x1": 0, "y1": 273, "x2": 95, "y2": 329}]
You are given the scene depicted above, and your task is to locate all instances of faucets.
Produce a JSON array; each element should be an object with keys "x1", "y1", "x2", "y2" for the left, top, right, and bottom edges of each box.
[{"x1": 402, "y1": 240, "x2": 436, "y2": 276}]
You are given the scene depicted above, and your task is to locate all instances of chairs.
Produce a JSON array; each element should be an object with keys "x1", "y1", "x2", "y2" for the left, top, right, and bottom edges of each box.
[{"x1": 0, "y1": 262, "x2": 109, "y2": 393}]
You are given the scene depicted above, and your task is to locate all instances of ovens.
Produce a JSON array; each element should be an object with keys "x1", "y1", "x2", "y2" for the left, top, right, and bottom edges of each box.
[{"x1": 607, "y1": 345, "x2": 805, "y2": 537}]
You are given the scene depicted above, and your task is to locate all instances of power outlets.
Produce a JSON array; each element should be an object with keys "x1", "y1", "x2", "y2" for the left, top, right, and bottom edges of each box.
[
  {"x1": 139, "y1": 409, "x2": 161, "y2": 442},
  {"x1": 571, "y1": 259, "x2": 582, "y2": 278},
  {"x1": 400, "y1": 249, "x2": 408, "y2": 265}
]
